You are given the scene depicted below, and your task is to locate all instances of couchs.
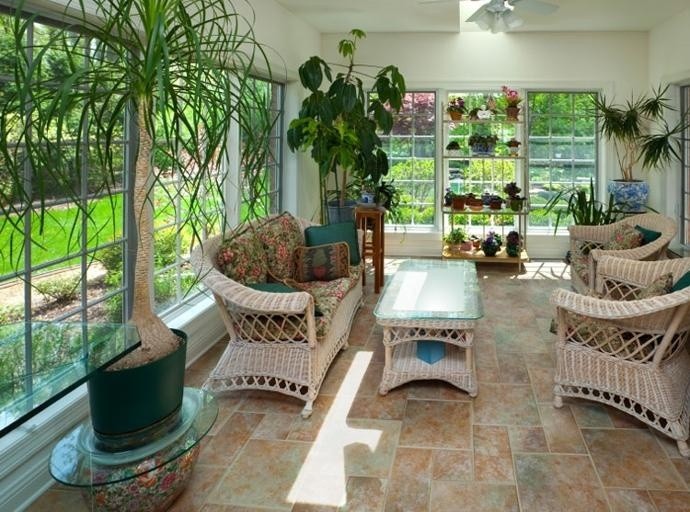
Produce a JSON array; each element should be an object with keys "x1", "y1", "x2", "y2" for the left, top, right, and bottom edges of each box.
[{"x1": 191, "y1": 214, "x2": 365, "y2": 419}]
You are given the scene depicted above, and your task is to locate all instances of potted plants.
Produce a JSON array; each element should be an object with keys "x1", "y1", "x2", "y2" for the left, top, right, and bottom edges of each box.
[
  {"x1": 1, "y1": 2, "x2": 275, "y2": 454},
  {"x1": 582, "y1": 81, "x2": 690, "y2": 214},
  {"x1": 446, "y1": 92, "x2": 524, "y2": 258},
  {"x1": 286, "y1": 27, "x2": 402, "y2": 225}
]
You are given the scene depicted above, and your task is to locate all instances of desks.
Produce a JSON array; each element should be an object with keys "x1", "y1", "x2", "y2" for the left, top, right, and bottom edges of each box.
[{"x1": 355, "y1": 208, "x2": 385, "y2": 294}]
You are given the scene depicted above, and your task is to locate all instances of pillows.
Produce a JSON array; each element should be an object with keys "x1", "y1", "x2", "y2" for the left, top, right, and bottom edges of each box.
[{"x1": 217, "y1": 211, "x2": 362, "y2": 317}]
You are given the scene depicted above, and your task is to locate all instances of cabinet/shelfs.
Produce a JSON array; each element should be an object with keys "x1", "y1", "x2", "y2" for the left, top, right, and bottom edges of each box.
[{"x1": 441, "y1": 103, "x2": 530, "y2": 273}]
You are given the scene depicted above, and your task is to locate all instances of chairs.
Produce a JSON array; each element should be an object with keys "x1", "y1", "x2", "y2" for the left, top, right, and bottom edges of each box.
[{"x1": 552, "y1": 212, "x2": 690, "y2": 456}]
